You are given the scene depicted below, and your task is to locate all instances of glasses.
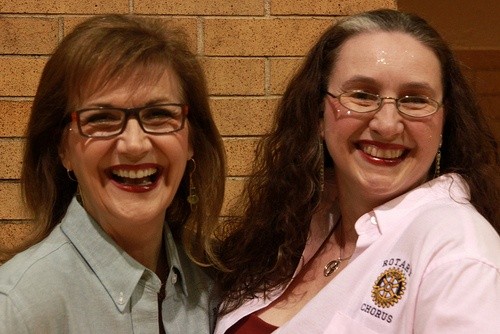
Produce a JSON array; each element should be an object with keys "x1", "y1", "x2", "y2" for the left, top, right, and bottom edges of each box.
[
  {"x1": 60, "y1": 103, "x2": 191, "y2": 139},
  {"x1": 324, "y1": 90, "x2": 447, "y2": 118}
]
